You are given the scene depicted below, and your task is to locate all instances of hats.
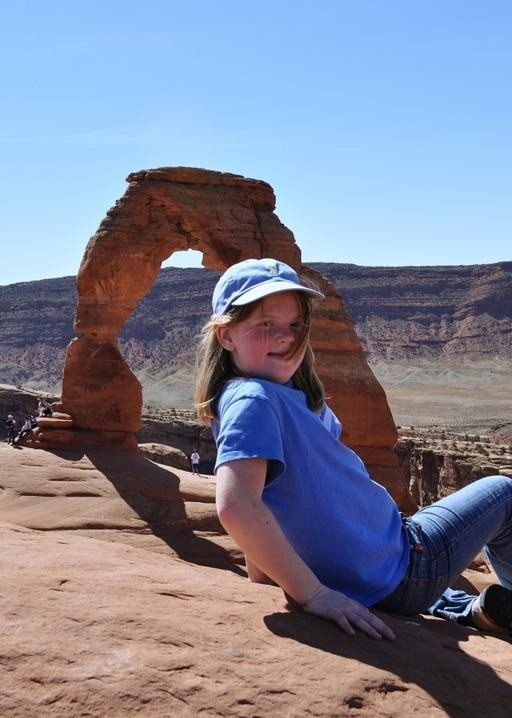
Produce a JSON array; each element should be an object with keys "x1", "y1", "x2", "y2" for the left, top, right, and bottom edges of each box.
[{"x1": 211, "y1": 258, "x2": 325, "y2": 319}]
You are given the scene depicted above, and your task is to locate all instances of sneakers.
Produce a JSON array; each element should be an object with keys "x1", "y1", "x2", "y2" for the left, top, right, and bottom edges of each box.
[{"x1": 470, "y1": 584, "x2": 512, "y2": 636}]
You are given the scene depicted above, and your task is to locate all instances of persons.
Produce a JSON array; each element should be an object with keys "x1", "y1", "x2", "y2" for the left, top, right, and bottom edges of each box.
[
  {"x1": 191, "y1": 448, "x2": 201, "y2": 475},
  {"x1": 191, "y1": 257, "x2": 510, "y2": 644},
  {"x1": 6, "y1": 397, "x2": 54, "y2": 445}
]
